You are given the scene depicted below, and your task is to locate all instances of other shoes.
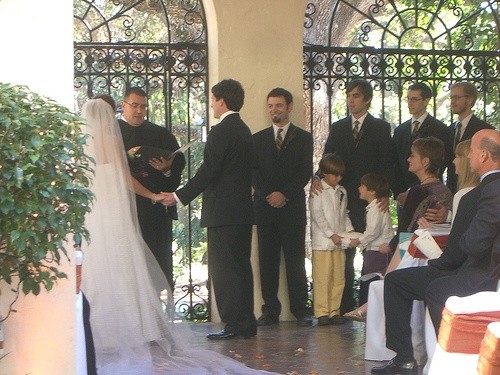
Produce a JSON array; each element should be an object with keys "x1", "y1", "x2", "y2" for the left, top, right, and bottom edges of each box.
[
  {"x1": 343, "y1": 309, "x2": 367, "y2": 322},
  {"x1": 331, "y1": 314, "x2": 345, "y2": 324},
  {"x1": 318, "y1": 316, "x2": 331, "y2": 326}
]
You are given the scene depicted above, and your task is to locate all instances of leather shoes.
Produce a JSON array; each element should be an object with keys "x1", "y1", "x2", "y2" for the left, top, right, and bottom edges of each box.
[
  {"x1": 207, "y1": 329, "x2": 249, "y2": 340},
  {"x1": 256, "y1": 313, "x2": 278, "y2": 325},
  {"x1": 371, "y1": 356, "x2": 418, "y2": 375},
  {"x1": 297, "y1": 316, "x2": 312, "y2": 327}
]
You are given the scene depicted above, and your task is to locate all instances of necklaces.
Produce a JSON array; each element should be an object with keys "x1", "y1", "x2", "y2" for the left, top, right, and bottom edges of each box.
[{"x1": 419, "y1": 176, "x2": 436, "y2": 184}]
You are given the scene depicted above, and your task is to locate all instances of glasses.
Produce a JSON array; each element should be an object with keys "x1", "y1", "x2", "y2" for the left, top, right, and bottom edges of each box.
[
  {"x1": 405, "y1": 96, "x2": 424, "y2": 103},
  {"x1": 450, "y1": 96, "x2": 466, "y2": 100},
  {"x1": 125, "y1": 102, "x2": 147, "y2": 109}
]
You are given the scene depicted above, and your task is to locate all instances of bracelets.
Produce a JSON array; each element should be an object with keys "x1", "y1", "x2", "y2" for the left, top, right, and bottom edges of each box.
[{"x1": 152, "y1": 193, "x2": 156, "y2": 205}]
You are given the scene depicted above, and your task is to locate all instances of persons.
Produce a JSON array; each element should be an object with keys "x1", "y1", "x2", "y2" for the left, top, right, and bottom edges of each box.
[
  {"x1": 448, "y1": 82, "x2": 496, "y2": 195},
  {"x1": 251, "y1": 88, "x2": 313, "y2": 327},
  {"x1": 350, "y1": 173, "x2": 395, "y2": 307},
  {"x1": 309, "y1": 79, "x2": 392, "y2": 316},
  {"x1": 117, "y1": 87, "x2": 186, "y2": 300},
  {"x1": 308, "y1": 153, "x2": 355, "y2": 326},
  {"x1": 343, "y1": 136, "x2": 453, "y2": 322},
  {"x1": 159, "y1": 79, "x2": 258, "y2": 340},
  {"x1": 80, "y1": 95, "x2": 168, "y2": 346},
  {"x1": 371, "y1": 128, "x2": 500, "y2": 375},
  {"x1": 417, "y1": 140, "x2": 480, "y2": 230},
  {"x1": 393, "y1": 83, "x2": 448, "y2": 199}
]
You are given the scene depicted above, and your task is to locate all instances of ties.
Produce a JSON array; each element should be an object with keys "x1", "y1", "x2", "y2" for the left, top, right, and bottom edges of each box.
[
  {"x1": 352, "y1": 121, "x2": 359, "y2": 145},
  {"x1": 275, "y1": 128, "x2": 283, "y2": 151},
  {"x1": 455, "y1": 123, "x2": 462, "y2": 146},
  {"x1": 412, "y1": 121, "x2": 420, "y2": 139}
]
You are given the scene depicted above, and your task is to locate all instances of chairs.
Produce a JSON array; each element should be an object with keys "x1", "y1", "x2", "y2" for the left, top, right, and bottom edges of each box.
[{"x1": 364, "y1": 222, "x2": 500, "y2": 375}]
